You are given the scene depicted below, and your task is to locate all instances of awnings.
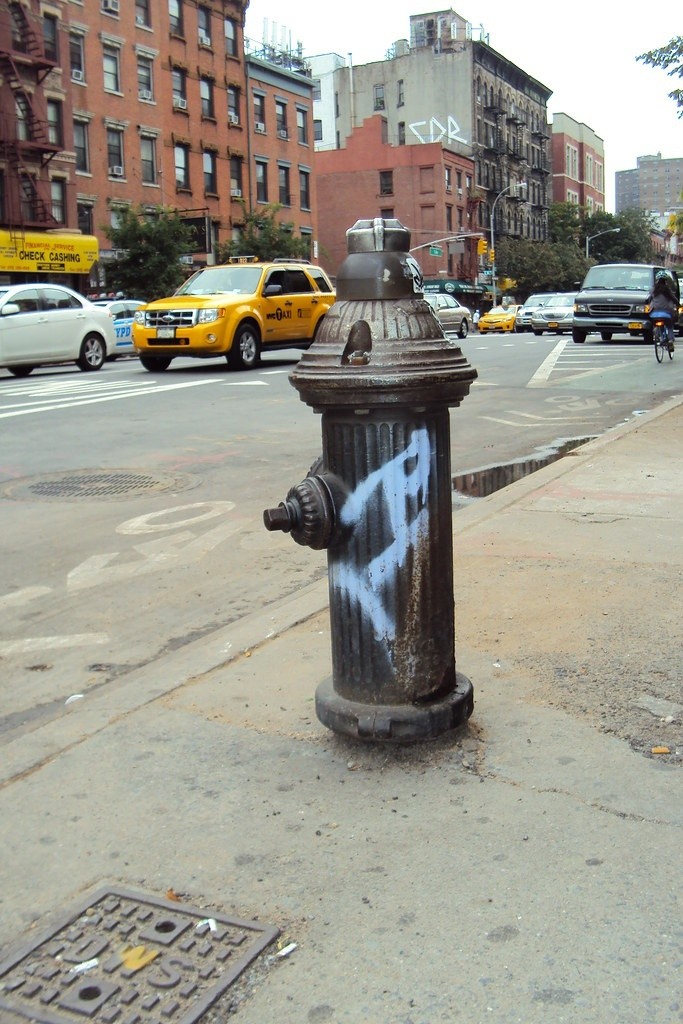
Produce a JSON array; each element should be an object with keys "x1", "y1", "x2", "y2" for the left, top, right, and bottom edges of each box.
[{"x1": 423, "y1": 280, "x2": 501, "y2": 297}]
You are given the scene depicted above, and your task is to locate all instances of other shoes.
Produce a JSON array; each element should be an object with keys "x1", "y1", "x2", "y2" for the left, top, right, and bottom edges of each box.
[
  {"x1": 668, "y1": 340, "x2": 674, "y2": 352},
  {"x1": 653, "y1": 334, "x2": 659, "y2": 341}
]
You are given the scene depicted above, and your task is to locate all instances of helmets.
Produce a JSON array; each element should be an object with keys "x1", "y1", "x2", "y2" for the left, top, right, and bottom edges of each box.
[{"x1": 655, "y1": 270, "x2": 667, "y2": 280}]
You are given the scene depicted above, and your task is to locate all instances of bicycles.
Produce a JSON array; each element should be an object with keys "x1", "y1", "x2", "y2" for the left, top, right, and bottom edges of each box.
[{"x1": 652, "y1": 318, "x2": 675, "y2": 363}]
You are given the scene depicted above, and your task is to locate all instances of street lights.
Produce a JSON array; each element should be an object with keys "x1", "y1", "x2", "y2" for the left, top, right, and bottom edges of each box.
[
  {"x1": 584, "y1": 227, "x2": 621, "y2": 258},
  {"x1": 489, "y1": 182, "x2": 528, "y2": 307}
]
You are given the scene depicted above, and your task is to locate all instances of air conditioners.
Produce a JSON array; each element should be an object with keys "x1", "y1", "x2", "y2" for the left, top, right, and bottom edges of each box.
[
  {"x1": 70, "y1": 68, "x2": 83, "y2": 81},
  {"x1": 109, "y1": 166, "x2": 123, "y2": 177},
  {"x1": 102, "y1": 0, "x2": 120, "y2": 13},
  {"x1": 255, "y1": 123, "x2": 264, "y2": 132},
  {"x1": 278, "y1": 130, "x2": 287, "y2": 137},
  {"x1": 175, "y1": 99, "x2": 187, "y2": 109},
  {"x1": 228, "y1": 114, "x2": 238, "y2": 125},
  {"x1": 138, "y1": 90, "x2": 151, "y2": 100},
  {"x1": 113, "y1": 251, "x2": 125, "y2": 259},
  {"x1": 182, "y1": 256, "x2": 193, "y2": 264},
  {"x1": 231, "y1": 189, "x2": 241, "y2": 197},
  {"x1": 198, "y1": 36, "x2": 210, "y2": 46}
]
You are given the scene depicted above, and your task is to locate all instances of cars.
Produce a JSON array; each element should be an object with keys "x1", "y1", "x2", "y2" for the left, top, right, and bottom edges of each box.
[
  {"x1": 128, "y1": 253, "x2": 336, "y2": 373},
  {"x1": 0, "y1": 282, "x2": 119, "y2": 377},
  {"x1": 421, "y1": 292, "x2": 473, "y2": 339},
  {"x1": 477, "y1": 295, "x2": 524, "y2": 335},
  {"x1": 84, "y1": 289, "x2": 149, "y2": 362},
  {"x1": 514, "y1": 289, "x2": 580, "y2": 335}
]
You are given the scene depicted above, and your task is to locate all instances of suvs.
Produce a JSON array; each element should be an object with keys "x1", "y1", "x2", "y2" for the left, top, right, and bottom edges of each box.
[{"x1": 571, "y1": 261, "x2": 683, "y2": 346}]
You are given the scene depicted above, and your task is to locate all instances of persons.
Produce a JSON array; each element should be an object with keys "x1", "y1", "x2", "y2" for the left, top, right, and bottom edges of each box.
[
  {"x1": 646, "y1": 270, "x2": 681, "y2": 352},
  {"x1": 473, "y1": 310, "x2": 480, "y2": 325}
]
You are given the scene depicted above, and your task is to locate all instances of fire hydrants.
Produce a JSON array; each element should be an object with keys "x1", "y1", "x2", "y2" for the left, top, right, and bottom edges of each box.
[{"x1": 260, "y1": 218, "x2": 481, "y2": 744}]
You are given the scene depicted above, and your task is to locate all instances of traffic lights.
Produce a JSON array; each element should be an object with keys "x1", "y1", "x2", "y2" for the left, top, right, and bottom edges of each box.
[
  {"x1": 490, "y1": 248, "x2": 495, "y2": 262},
  {"x1": 480, "y1": 239, "x2": 488, "y2": 254}
]
